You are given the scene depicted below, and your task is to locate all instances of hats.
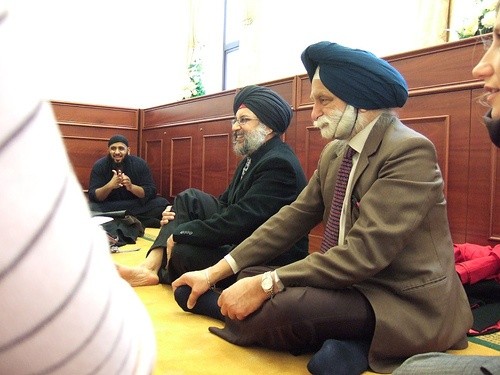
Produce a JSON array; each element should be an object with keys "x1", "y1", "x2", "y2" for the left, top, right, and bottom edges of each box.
[
  {"x1": 233, "y1": 84, "x2": 294, "y2": 136},
  {"x1": 107, "y1": 135, "x2": 130, "y2": 147},
  {"x1": 299, "y1": 41, "x2": 409, "y2": 110}
]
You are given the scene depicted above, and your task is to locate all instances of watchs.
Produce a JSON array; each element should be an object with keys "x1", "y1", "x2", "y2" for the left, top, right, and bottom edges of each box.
[{"x1": 261, "y1": 271, "x2": 275, "y2": 299}]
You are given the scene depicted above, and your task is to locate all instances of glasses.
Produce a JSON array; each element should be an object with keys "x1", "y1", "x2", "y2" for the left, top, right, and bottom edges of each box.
[{"x1": 233, "y1": 117, "x2": 259, "y2": 125}]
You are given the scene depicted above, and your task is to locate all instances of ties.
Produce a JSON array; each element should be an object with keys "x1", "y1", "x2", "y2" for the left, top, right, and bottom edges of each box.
[
  {"x1": 318, "y1": 145, "x2": 356, "y2": 254},
  {"x1": 238, "y1": 155, "x2": 252, "y2": 180}
]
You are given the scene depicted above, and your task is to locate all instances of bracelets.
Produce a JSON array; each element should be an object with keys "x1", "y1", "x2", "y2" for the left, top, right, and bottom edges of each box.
[{"x1": 205, "y1": 269, "x2": 215, "y2": 289}]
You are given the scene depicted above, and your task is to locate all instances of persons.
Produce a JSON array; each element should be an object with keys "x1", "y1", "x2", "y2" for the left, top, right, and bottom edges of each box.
[
  {"x1": 114, "y1": 85, "x2": 311, "y2": 288},
  {"x1": 171, "y1": 40, "x2": 474, "y2": 375},
  {"x1": 87, "y1": 135, "x2": 171, "y2": 228}
]
